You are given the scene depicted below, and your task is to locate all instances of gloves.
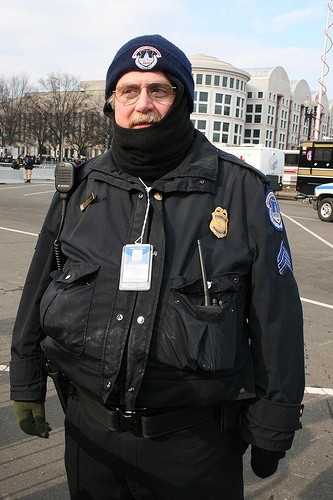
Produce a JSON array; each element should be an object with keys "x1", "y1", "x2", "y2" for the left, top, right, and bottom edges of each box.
[
  {"x1": 250, "y1": 445, "x2": 286, "y2": 478},
  {"x1": 13, "y1": 400, "x2": 52, "y2": 438}
]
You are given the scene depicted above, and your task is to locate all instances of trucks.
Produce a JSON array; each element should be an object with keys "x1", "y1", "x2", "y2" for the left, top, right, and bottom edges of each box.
[{"x1": 217, "y1": 146, "x2": 284, "y2": 184}]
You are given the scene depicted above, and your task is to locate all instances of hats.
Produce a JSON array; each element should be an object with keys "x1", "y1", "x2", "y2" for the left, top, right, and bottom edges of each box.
[{"x1": 105, "y1": 35, "x2": 194, "y2": 116}]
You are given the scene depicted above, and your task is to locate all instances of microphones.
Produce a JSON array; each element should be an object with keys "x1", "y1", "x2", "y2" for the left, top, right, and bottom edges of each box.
[{"x1": 54, "y1": 160, "x2": 77, "y2": 200}]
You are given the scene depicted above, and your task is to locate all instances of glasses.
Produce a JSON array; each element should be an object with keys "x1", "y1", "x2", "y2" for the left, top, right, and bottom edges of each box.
[{"x1": 113, "y1": 81, "x2": 176, "y2": 105}]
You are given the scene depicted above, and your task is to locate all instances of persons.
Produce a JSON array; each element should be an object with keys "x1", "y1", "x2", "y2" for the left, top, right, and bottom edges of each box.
[
  {"x1": 11, "y1": 153, "x2": 87, "y2": 183},
  {"x1": 9, "y1": 34, "x2": 305, "y2": 500}
]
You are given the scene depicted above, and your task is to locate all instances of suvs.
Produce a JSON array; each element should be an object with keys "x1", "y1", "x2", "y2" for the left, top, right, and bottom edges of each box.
[{"x1": 312, "y1": 182, "x2": 333, "y2": 221}]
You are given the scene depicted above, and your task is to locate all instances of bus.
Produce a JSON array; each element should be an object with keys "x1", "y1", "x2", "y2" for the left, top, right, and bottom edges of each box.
[
  {"x1": 296, "y1": 140, "x2": 333, "y2": 194},
  {"x1": 283, "y1": 149, "x2": 300, "y2": 184}
]
